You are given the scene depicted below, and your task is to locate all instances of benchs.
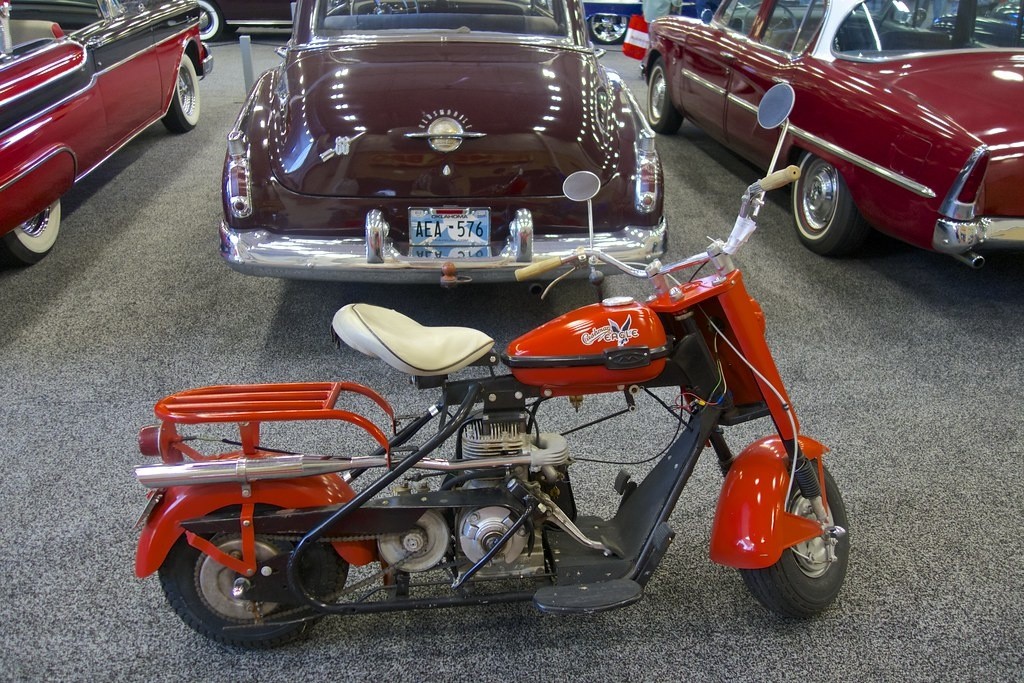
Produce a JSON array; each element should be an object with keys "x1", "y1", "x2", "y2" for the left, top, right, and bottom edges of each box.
[{"x1": 323, "y1": 14, "x2": 559, "y2": 36}]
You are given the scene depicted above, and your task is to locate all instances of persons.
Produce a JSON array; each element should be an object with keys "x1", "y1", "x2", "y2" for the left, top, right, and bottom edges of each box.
[{"x1": 640, "y1": 0, "x2": 683, "y2": 74}]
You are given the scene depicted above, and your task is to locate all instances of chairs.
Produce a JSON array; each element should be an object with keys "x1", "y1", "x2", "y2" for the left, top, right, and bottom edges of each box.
[
  {"x1": 869, "y1": 30, "x2": 951, "y2": 51},
  {"x1": 9, "y1": 20, "x2": 64, "y2": 46}
]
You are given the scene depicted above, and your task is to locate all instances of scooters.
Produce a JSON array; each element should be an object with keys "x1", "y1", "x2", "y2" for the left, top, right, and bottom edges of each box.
[{"x1": 130, "y1": 80, "x2": 851, "y2": 652}]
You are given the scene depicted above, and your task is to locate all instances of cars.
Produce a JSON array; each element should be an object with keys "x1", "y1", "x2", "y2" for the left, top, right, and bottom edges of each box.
[
  {"x1": 928, "y1": 0, "x2": 1023, "y2": 51},
  {"x1": 639, "y1": 0, "x2": 1024, "y2": 270},
  {"x1": 0, "y1": 0, "x2": 210, "y2": 265},
  {"x1": 217, "y1": 0, "x2": 668, "y2": 284},
  {"x1": 577, "y1": 1, "x2": 732, "y2": 49},
  {"x1": 213, "y1": 0, "x2": 346, "y2": 39}
]
